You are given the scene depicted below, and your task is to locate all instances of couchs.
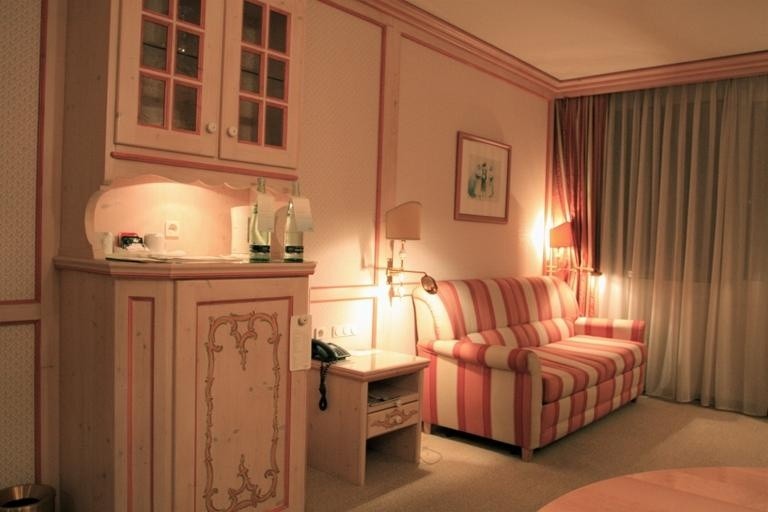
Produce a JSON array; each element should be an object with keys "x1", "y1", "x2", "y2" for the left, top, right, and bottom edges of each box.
[{"x1": 412, "y1": 276, "x2": 648, "y2": 461}]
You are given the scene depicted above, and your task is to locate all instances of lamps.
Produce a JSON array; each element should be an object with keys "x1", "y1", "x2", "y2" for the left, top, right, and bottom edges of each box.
[
  {"x1": 361, "y1": 201, "x2": 438, "y2": 294},
  {"x1": 543, "y1": 222, "x2": 603, "y2": 278}
]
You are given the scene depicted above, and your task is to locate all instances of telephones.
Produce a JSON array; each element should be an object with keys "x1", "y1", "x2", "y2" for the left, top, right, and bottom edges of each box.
[{"x1": 311, "y1": 339, "x2": 351, "y2": 411}]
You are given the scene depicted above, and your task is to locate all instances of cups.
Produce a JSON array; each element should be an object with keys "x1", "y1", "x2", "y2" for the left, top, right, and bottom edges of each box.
[{"x1": 143, "y1": 228, "x2": 165, "y2": 252}]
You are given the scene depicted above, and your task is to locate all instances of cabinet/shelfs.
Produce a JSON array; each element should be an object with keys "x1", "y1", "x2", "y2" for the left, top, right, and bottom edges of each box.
[
  {"x1": 59, "y1": 2, "x2": 306, "y2": 260},
  {"x1": 52, "y1": 261, "x2": 316, "y2": 512}
]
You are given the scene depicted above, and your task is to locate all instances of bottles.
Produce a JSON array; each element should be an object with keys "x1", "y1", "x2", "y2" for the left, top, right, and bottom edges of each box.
[
  {"x1": 283, "y1": 181, "x2": 304, "y2": 263},
  {"x1": 247, "y1": 176, "x2": 272, "y2": 263}
]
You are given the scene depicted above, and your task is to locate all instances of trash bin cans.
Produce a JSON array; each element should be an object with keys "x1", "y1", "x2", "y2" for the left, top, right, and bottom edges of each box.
[{"x1": 0, "y1": 484, "x2": 57, "y2": 512}]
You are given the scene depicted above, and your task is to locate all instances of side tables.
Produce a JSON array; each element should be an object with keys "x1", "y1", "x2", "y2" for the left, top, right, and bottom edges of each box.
[{"x1": 312, "y1": 349, "x2": 431, "y2": 485}]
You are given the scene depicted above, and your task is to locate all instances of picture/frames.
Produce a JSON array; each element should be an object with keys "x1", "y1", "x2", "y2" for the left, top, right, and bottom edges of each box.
[{"x1": 453, "y1": 130, "x2": 513, "y2": 225}]
